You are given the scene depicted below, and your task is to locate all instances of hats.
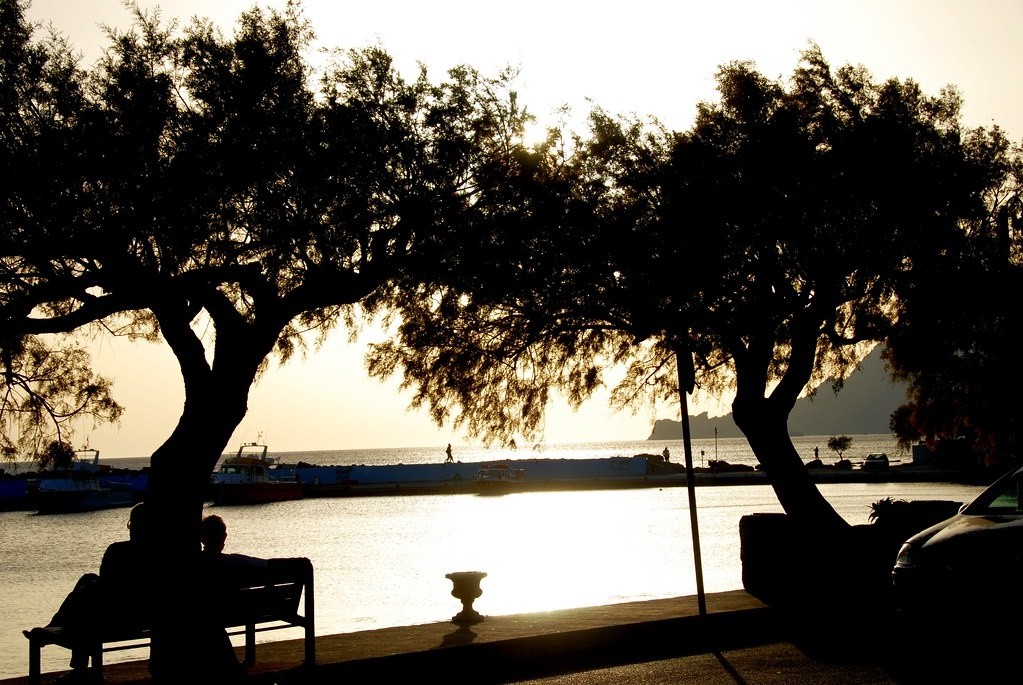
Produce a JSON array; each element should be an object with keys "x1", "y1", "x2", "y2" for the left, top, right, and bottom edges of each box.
[{"x1": 129, "y1": 502, "x2": 144, "y2": 523}]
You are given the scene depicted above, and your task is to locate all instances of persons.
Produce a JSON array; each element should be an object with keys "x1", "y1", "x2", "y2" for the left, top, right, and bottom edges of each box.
[
  {"x1": 200, "y1": 517, "x2": 305, "y2": 621},
  {"x1": 444, "y1": 444, "x2": 453, "y2": 462},
  {"x1": 813, "y1": 447, "x2": 819, "y2": 459},
  {"x1": 23, "y1": 504, "x2": 146, "y2": 684},
  {"x1": 663, "y1": 447, "x2": 670, "y2": 462}
]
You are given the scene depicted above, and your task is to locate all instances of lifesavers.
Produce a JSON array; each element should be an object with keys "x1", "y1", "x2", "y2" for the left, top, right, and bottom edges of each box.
[{"x1": 453, "y1": 473, "x2": 462, "y2": 481}]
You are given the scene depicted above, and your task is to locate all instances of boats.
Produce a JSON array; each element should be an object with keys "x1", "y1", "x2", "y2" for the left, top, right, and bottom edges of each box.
[
  {"x1": 211, "y1": 442, "x2": 303, "y2": 506},
  {"x1": 24, "y1": 469, "x2": 111, "y2": 514},
  {"x1": 471, "y1": 463, "x2": 530, "y2": 496}
]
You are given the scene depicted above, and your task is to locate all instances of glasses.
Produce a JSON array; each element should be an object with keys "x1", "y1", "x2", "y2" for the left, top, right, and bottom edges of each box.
[{"x1": 126, "y1": 519, "x2": 132, "y2": 529}]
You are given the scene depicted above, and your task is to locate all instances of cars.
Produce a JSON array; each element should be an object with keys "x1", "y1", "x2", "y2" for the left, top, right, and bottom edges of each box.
[{"x1": 862, "y1": 454, "x2": 889, "y2": 470}]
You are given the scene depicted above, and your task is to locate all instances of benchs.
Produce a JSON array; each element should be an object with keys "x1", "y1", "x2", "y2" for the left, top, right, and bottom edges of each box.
[
  {"x1": 221, "y1": 458, "x2": 266, "y2": 472},
  {"x1": 27, "y1": 552, "x2": 318, "y2": 675}
]
